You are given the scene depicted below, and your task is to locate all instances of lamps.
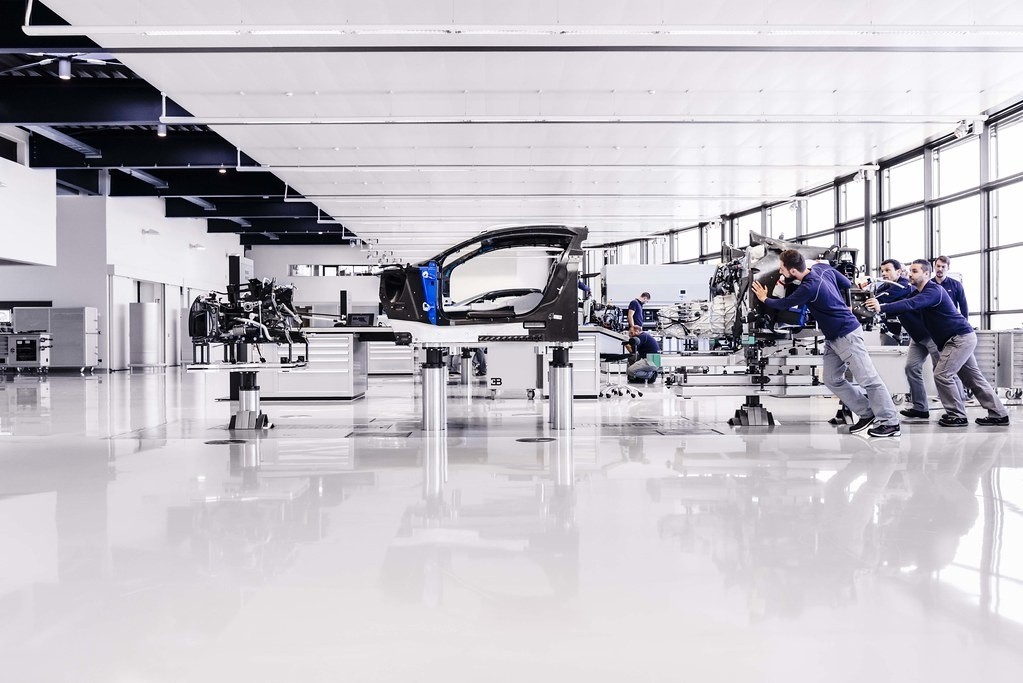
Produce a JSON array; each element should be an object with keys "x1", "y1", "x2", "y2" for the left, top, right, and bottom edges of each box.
[
  {"x1": 58, "y1": 60, "x2": 71, "y2": 80},
  {"x1": 157, "y1": 124, "x2": 167, "y2": 137}
]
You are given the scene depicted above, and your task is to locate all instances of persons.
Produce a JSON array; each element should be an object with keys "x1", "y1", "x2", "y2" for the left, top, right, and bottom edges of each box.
[
  {"x1": 628, "y1": 292, "x2": 651, "y2": 326},
  {"x1": 865, "y1": 259, "x2": 1010, "y2": 426},
  {"x1": 752, "y1": 250, "x2": 901, "y2": 437},
  {"x1": 876, "y1": 259, "x2": 966, "y2": 419},
  {"x1": 931, "y1": 256, "x2": 968, "y2": 320},
  {"x1": 625, "y1": 325, "x2": 660, "y2": 385}
]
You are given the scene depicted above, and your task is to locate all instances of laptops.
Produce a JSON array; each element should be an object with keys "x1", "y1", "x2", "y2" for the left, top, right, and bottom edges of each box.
[{"x1": 345, "y1": 313, "x2": 374, "y2": 326}]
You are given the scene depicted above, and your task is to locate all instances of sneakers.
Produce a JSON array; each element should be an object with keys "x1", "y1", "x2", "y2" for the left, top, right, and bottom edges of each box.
[
  {"x1": 975, "y1": 415, "x2": 1010, "y2": 426},
  {"x1": 867, "y1": 423, "x2": 901, "y2": 437},
  {"x1": 849, "y1": 413, "x2": 876, "y2": 433},
  {"x1": 938, "y1": 417, "x2": 968, "y2": 426}
]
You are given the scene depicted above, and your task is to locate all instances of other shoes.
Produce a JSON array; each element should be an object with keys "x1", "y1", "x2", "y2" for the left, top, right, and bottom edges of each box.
[
  {"x1": 942, "y1": 414, "x2": 948, "y2": 418},
  {"x1": 900, "y1": 407, "x2": 929, "y2": 418},
  {"x1": 647, "y1": 371, "x2": 657, "y2": 384}
]
